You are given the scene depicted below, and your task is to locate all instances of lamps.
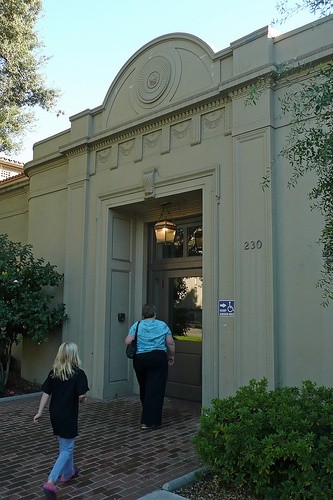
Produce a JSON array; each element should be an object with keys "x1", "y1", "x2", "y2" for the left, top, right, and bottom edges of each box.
[{"x1": 154, "y1": 202, "x2": 177, "y2": 246}]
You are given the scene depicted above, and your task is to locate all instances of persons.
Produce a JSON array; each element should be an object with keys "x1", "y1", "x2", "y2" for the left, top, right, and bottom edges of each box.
[
  {"x1": 125, "y1": 304, "x2": 175, "y2": 429},
  {"x1": 34, "y1": 341, "x2": 89, "y2": 500}
]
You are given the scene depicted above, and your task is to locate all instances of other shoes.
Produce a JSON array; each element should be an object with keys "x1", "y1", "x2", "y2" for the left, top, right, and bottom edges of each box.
[
  {"x1": 142, "y1": 423, "x2": 155, "y2": 429},
  {"x1": 60, "y1": 466, "x2": 81, "y2": 482},
  {"x1": 43, "y1": 484, "x2": 57, "y2": 500}
]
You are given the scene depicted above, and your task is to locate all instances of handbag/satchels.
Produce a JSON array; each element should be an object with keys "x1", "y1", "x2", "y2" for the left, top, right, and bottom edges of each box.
[{"x1": 126, "y1": 320, "x2": 140, "y2": 359}]
